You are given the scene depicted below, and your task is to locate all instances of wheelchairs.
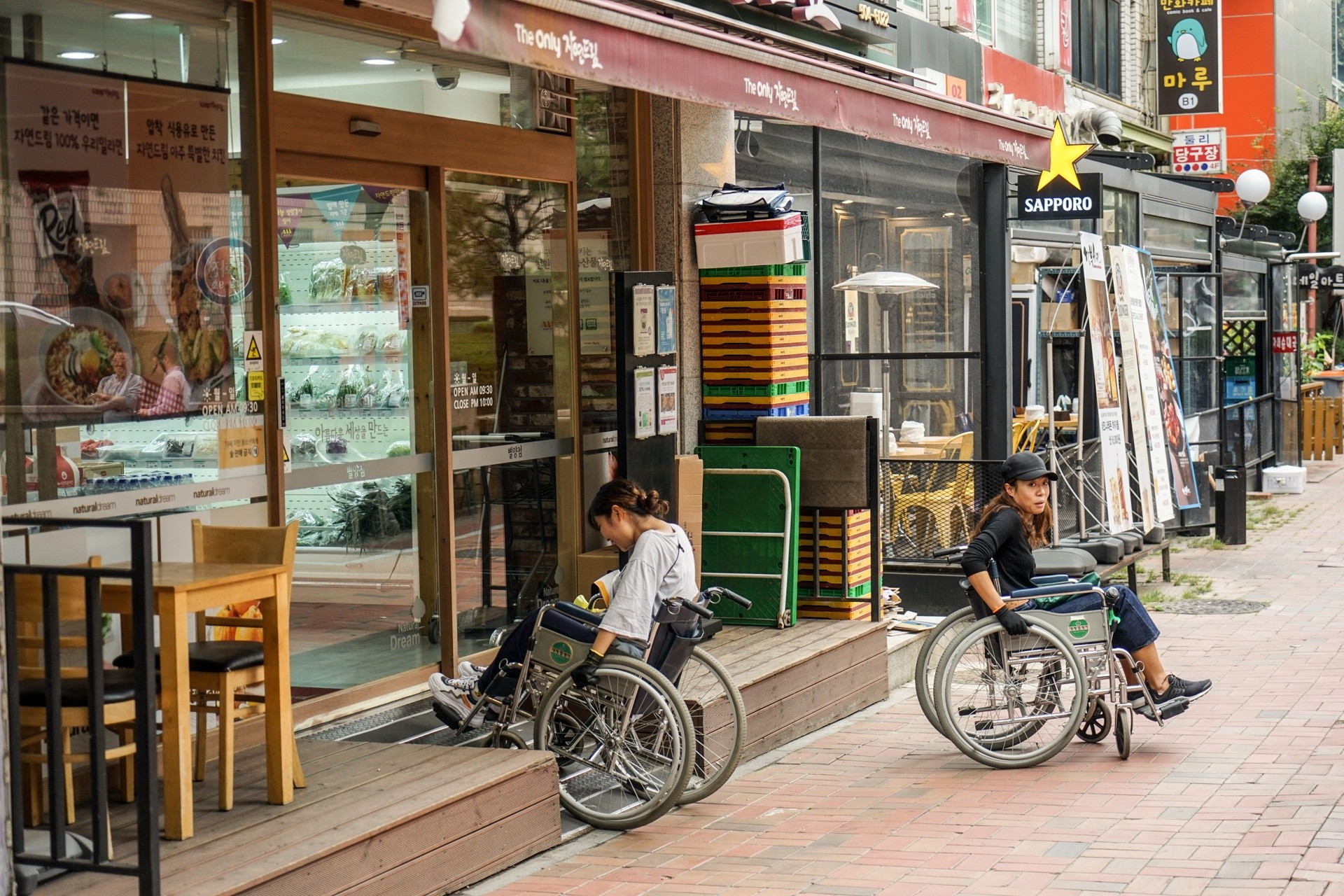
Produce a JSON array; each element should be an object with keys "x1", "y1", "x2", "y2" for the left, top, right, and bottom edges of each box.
[
  {"x1": 914, "y1": 547, "x2": 1188, "y2": 768},
  {"x1": 434, "y1": 587, "x2": 753, "y2": 832}
]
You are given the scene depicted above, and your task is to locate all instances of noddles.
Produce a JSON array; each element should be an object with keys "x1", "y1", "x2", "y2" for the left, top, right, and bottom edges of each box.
[{"x1": 45, "y1": 323, "x2": 120, "y2": 405}]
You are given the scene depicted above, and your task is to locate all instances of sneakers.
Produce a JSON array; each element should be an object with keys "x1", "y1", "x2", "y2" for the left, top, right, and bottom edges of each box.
[
  {"x1": 1150, "y1": 672, "x2": 1212, "y2": 713},
  {"x1": 428, "y1": 672, "x2": 490, "y2": 728},
  {"x1": 1126, "y1": 680, "x2": 1151, "y2": 708},
  {"x1": 458, "y1": 661, "x2": 489, "y2": 683}
]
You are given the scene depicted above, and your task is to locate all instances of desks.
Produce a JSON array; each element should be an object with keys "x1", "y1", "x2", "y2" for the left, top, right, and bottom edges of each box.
[
  {"x1": 1013, "y1": 414, "x2": 1079, "y2": 504},
  {"x1": 897, "y1": 436, "x2": 962, "y2": 490},
  {"x1": 880, "y1": 446, "x2": 946, "y2": 494},
  {"x1": 101, "y1": 560, "x2": 293, "y2": 840}
]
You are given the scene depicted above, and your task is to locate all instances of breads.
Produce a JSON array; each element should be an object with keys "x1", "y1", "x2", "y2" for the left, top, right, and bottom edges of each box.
[{"x1": 343, "y1": 267, "x2": 397, "y2": 302}]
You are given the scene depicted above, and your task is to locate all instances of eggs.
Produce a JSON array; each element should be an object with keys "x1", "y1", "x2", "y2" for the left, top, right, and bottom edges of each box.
[{"x1": 80, "y1": 347, "x2": 101, "y2": 370}]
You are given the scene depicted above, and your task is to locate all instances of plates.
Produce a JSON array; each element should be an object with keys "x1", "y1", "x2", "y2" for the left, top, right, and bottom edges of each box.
[{"x1": 195, "y1": 236, "x2": 253, "y2": 305}]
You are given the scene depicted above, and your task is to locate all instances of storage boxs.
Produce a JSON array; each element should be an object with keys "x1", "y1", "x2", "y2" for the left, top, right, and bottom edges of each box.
[
  {"x1": 76, "y1": 462, "x2": 124, "y2": 488},
  {"x1": 1261, "y1": 465, "x2": 1307, "y2": 494},
  {"x1": 675, "y1": 213, "x2": 883, "y2": 621},
  {"x1": 1223, "y1": 355, "x2": 1257, "y2": 420},
  {"x1": 1040, "y1": 289, "x2": 1078, "y2": 331},
  {"x1": 576, "y1": 545, "x2": 620, "y2": 608},
  {"x1": 31, "y1": 426, "x2": 81, "y2": 463}
]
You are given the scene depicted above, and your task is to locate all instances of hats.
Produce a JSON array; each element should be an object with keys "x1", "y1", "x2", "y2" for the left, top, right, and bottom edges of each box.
[{"x1": 1001, "y1": 452, "x2": 1058, "y2": 484}]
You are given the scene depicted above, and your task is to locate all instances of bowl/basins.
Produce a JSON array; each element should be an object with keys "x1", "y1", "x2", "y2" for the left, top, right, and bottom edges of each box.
[
  {"x1": 1025, "y1": 405, "x2": 1045, "y2": 422},
  {"x1": 95, "y1": 431, "x2": 217, "y2": 460},
  {"x1": 37, "y1": 306, "x2": 133, "y2": 407}
]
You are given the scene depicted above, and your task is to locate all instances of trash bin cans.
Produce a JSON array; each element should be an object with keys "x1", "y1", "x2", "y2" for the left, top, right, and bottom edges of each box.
[{"x1": 1215, "y1": 464, "x2": 1247, "y2": 545}]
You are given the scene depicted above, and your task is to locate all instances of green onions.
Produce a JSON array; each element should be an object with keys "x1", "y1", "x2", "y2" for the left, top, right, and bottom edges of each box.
[{"x1": 326, "y1": 478, "x2": 411, "y2": 553}]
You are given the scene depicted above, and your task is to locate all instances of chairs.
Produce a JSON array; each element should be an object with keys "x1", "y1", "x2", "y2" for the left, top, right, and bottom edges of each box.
[
  {"x1": 880, "y1": 416, "x2": 1040, "y2": 559},
  {"x1": 111, "y1": 519, "x2": 307, "y2": 810},
  {"x1": 6, "y1": 556, "x2": 163, "y2": 858}
]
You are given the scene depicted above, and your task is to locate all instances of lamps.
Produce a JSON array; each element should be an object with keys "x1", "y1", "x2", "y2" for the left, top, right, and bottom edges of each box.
[
  {"x1": 1281, "y1": 191, "x2": 1327, "y2": 260},
  {"x1": 831, "y1": 253, "x2": 941, "y2": 433},
  {"x1": 1219, "y1": 169, "x2": 1271, "y2": 249}
]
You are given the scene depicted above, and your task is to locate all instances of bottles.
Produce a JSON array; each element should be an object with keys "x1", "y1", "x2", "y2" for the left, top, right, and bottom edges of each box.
[{"x1": 84, "y1": 473, "x2": 195, "y2": 511}]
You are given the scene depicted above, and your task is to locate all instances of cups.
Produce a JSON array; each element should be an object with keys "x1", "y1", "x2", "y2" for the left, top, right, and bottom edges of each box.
[
  {"x1": 1062, "y1": 404, "x2": 1072, "y2": 410},
  {"x1": 102, "y1": 272, "x2": 146, "y2": 317},
  {"x1": 1069, "y1": 413, "x2": 1078, "y2": 421}
]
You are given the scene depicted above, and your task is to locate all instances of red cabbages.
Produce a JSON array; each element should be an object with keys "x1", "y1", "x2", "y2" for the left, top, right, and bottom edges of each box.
[{"x1": 325, "y1": 437, "x2": 347, "y2": 454}]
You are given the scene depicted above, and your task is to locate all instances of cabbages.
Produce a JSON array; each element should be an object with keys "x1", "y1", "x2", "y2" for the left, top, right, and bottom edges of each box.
[{"x1": 387, "y1": 441, "x2": 411, "y2": 456}]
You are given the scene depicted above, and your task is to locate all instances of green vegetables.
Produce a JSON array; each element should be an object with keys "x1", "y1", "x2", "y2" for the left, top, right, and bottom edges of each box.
[
  {"x1": 307, "y1": 258, "x2": 344, "y2": 305},
  {"x1": 287, "y1": 378, "x2": 365, "y2": 408},
  {"x1": 279, "y1": 282, "x2": 292, "y2": 307},
  {"x1": 90, "y1": 330, "x2": 111, "y2": 371}
]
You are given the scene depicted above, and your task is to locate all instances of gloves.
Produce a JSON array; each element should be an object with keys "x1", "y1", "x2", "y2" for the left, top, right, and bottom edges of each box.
[
  {"x1": 993, "y1": 602, "x2": 1034, "y2": 635},
  {"x1": 571, "y1": 648, "x2": 605, "y2": 689}
]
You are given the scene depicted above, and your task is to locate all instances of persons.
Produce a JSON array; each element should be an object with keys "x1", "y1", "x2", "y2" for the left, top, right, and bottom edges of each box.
[
  {"x1": 91, "y1": 341, "x2": 190, "y2": 422},
  {"x1": 960, "y1": 451, "x2": 1212, "y2": 712},
  {"x1": 428, "y1": 479, "x2": 696, "y2": 729}
]
